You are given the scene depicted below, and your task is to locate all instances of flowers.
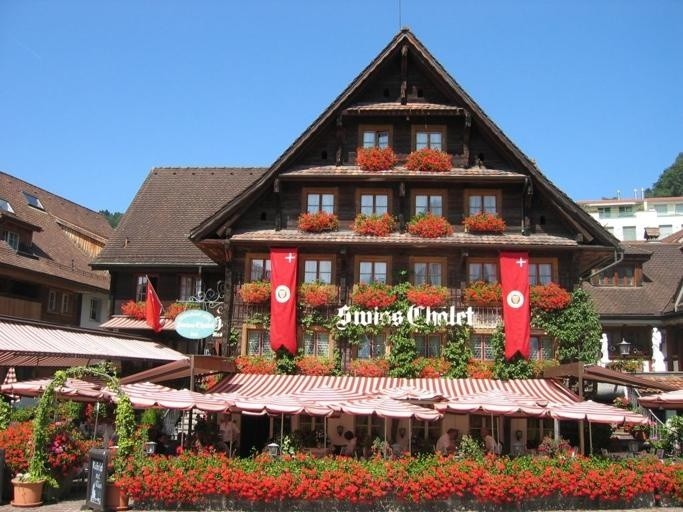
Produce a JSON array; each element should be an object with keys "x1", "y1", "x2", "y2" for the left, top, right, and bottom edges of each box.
[
  {"x1": 236, "y1": 278, "x2": 572, "y2": 314},
  {"x1": 108, "y1": 432, "x2": 682, "y2": 505},
  {"x1": 120, "y1": 299, "x2": 147, "y2": 320},
  {"x1": 234, "y1": 352, "x2": 495, "y2": 378},
  {"x1": 356, "y1": 145, "x2": 455, "y2": 173},
  {"x1": 16, "y1": 471, "x2": 41, "y2": 481},
  {"x1": 296, "y1": 210, "x2": 509, "y2": 239},
  {"x1": 1, "y1": 413, "x2": 106, "y2": 482},
  {"x1": 161, "y1": 302, "x2": 185, "y2": 320}
]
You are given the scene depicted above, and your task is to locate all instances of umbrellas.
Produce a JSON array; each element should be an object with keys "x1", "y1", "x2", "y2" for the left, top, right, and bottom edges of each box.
[{"x1": 636, "y1": 388, "x2": 683, "y2": 417}]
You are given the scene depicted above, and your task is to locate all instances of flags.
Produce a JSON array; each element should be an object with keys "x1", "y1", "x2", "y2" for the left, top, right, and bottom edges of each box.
[{"x1": 144, "y1": 282, "x2": 163, "y2": 332}]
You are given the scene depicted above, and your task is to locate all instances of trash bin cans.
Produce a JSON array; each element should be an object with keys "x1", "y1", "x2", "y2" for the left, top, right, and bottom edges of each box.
[{"x1": 86, "y1": 447, "x2": 107, "y2": 509}]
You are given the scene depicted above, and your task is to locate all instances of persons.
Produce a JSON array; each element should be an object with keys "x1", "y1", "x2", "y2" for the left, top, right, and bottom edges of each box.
[
  {"x1": 435, "y1": 429, "x2": 458, "y2": 457},
  {"x1": 481, "y1": 427, "x2": 499, "y2": 459},
  {"x1": 513, "y1": 430, "x2": 528, "y2": 458}
]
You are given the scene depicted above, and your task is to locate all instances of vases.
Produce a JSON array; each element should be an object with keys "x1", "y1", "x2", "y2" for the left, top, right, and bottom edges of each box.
[
  {"x1": 10, "y1": 477, "x2": 46, "y2": 507},
  {"x1": 104, "y1": 483, "x2": 128, "y2": 511}
]
